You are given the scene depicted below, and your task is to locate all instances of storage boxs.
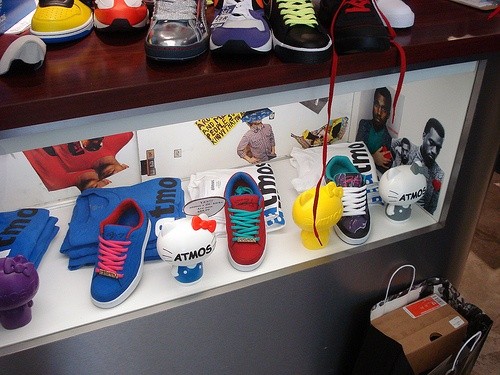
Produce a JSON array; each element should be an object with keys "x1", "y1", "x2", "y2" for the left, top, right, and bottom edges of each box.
[{"x1": 374, "y1": 294, "x2": 468, "y2": 375}]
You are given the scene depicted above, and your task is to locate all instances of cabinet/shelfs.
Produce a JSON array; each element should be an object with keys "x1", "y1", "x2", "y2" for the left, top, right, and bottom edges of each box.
[{"x1": 0, "y1": 0, "x2": 500, "y2": 375}]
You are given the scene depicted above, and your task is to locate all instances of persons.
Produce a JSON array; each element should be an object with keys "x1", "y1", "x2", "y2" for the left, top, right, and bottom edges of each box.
[
  {"x1": 237, "y1": 119, "x2": 276, "y2": 166},
  {"x1": 355, "y1": 86, "x2": 394, "y2": 174},
  {"x1": 391, "y1": 118, "x2": 445, "y2": 214},
  {"x1": 26, "y1": 131, "x2": 134, "y2": 195}
]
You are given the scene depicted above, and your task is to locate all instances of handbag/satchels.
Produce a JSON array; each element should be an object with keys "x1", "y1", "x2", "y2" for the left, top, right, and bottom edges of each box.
[{"x1": 351, "y1": 263, "x2": 493, "y2": 375}]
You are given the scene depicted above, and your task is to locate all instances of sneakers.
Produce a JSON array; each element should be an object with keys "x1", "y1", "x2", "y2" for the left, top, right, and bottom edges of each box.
[
  {"x1": 90, "y1": 197, "x2": 152, "y2": 309},
  {"x1": 208, "y1": 0, "x2": 273, "y2": 61},
  {"x1": 262, "y1": 0, "x2": 333, "y2": 62},
  {"x1": 144, "y1": 0, "x2": 211, "y2": 62},
  {"x1": 93, "y1": 0, "x2": 150, "y2": 32},
  {"x1": 323, "y1": 155, "x2": 371, "y2": 244},
  {"x1": 312, "y1": 0, "x2": 406, "y2": 246},
  {"x1": 224, "y1": 171, "x2": 268, "y2": 273}
]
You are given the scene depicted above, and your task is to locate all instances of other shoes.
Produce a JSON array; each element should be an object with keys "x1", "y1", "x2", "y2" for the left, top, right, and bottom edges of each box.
[
  {"x1": 29, "y1": 0, "x2": 94, "y2": 44},
  {"x1": 371, "y1": 0, "x2": 415, "y2": 29}
]
